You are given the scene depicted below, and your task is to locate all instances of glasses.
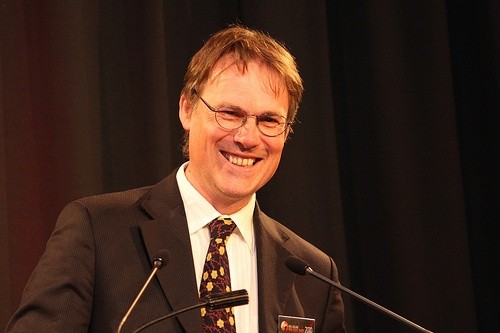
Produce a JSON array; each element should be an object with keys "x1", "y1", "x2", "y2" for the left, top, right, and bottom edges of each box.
[{"x1": 193, "y1": 88, "x2": 292, "y2": 137}]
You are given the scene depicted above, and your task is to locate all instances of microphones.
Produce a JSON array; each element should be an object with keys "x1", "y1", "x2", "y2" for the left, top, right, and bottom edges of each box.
[
  {"x1": 132, "y1": 289, "x2": 249, "y2": 333},
  {"x1": 116, "y1": 250, "x2": 171, "y2": 333},
  {"x1": 285, "y1": 255, "x2": 434, "y2": 333}
]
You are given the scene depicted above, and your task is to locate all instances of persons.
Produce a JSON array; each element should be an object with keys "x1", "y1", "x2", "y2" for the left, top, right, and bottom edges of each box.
[{"x1": 3, "y1": 26, "x2": 348, "y2": 333}]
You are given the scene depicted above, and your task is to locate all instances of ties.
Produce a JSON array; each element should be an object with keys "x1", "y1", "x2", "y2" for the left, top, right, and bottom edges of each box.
[{"x1": 198, "y1": 216, "x2": 237, "y2": 333}]
